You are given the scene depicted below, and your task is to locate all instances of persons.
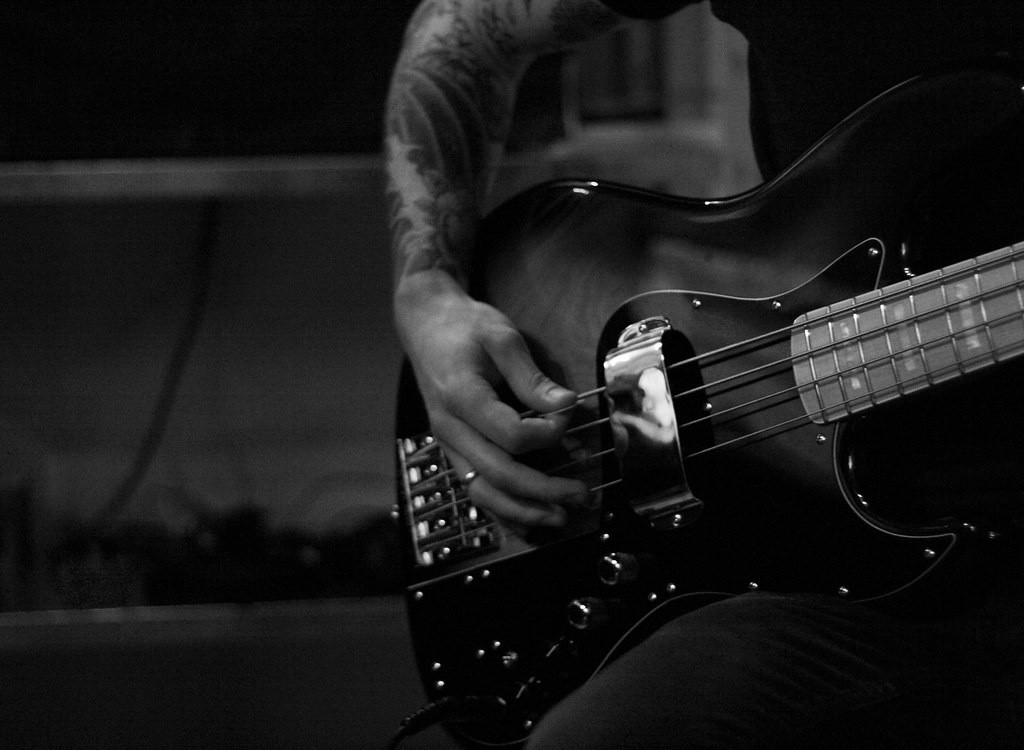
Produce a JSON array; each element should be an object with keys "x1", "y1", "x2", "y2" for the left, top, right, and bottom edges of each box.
[{"x1": 376, "y1": 1, "x2": 1024, "y2": 750}]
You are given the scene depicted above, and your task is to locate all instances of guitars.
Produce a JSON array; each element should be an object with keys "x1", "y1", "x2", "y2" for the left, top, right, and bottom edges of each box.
[{"x1": 391, "y1": 62, "x2": 1024, "y2": 750}]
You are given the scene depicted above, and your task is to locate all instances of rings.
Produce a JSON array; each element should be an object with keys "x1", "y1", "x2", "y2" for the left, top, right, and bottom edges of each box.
[{"x1": 461, "y1": 471, "x2": 478, "y2": 485}]
